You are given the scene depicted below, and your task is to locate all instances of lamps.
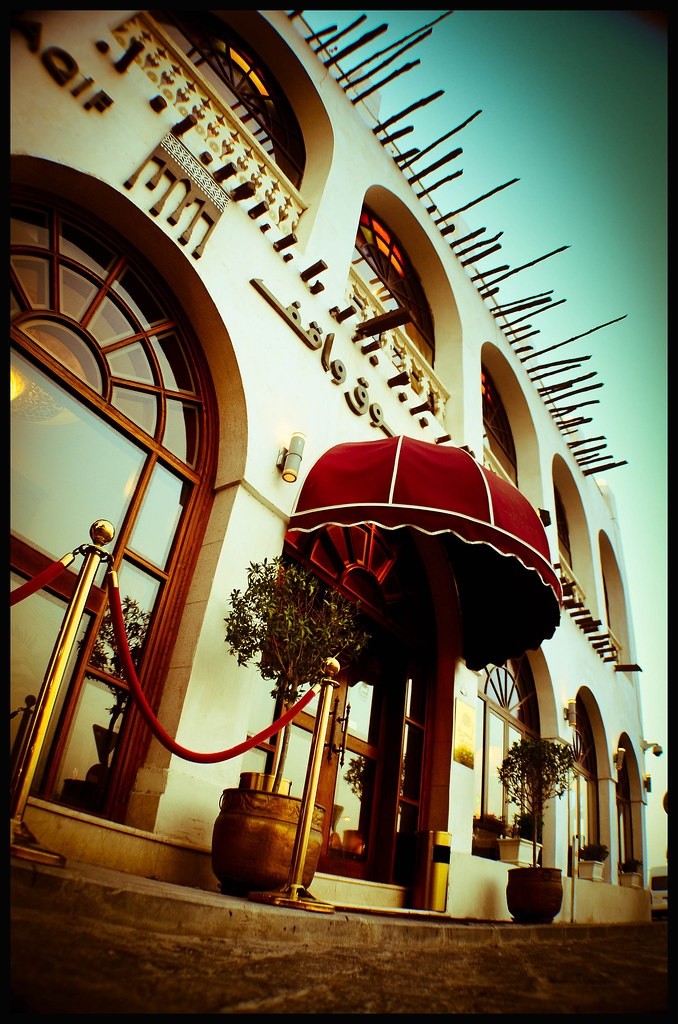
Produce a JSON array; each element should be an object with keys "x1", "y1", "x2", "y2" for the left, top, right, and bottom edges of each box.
[
  {"x1": 276, "y1": 432, "x2": 308, "y2": 484},
  {"x1": 614, "y1": 748, "x2": 626, "y2": 771},
  {"x1": 644, "y1": 774, "x2": 652, "y2": 792},
  {"x1": 564, "y1": 698, "x2": 577, "y2": 726}
]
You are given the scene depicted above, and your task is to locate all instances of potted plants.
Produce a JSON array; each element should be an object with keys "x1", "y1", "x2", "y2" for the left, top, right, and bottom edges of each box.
[
  {"x1": 208, "y1": 555, "x2": 372, "y2": 898},
  {"x1": 61, "y1": 594, "x2": 152, "y2": 811},
  {"x1": 578, "y1": 843, "x2": 610, "y2": 882},
  {"x1": 618, "y1": 858, "x2": 643, "y2": 889},
  {"x1": 496, "y1": 738, "x2": 582, "y2": 924},
  {"x1": 497, "y1": 812, "x2": 546, "y2": 867},
  {"x1": 471, "y1": 813, "x2": 508, "y2": 860}
]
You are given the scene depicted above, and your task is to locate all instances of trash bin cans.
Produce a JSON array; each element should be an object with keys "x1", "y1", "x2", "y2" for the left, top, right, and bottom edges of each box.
[{"x1": 239, "y1": 771, "x2": 292, "y2": 796}]
